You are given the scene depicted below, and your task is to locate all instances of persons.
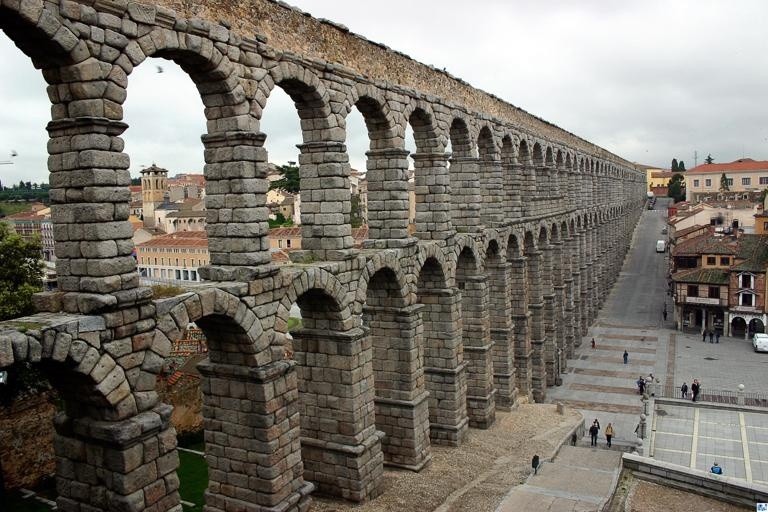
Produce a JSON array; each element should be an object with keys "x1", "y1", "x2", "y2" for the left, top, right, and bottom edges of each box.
[
  {"x1": 680, "y1": 381, "x2": 687, "y2": 398},
  {"x1": 648, "y1": 373, "x2": 655, "y2": 380},
  {"x1": 692, "y1": 378, "x2": 701, "y2": 401},
  {"x1": 590, "y1": 337, "x2": 597, "y2": 351},
  {"x1": 638, "y1": 376, "x2": 642, "y2": 387},
  {"x1": 604, "y1": 422, "x2": 615, "y2": 447},
  {"x1": 622, "y1": 349, "x2": 628, "y2": 364},
  {"x1": 572, "y1": 431, "x2": 578, "y2": 446},
  {"x1": 663, "y1": 308, "x2": 668, "y2": 320},
  {"x1": 709, "y1": 331, "x2": 714, "y2": 343},
  {"x1": 710, "y1": 461, "x2": 723, "y2": 474},
  {"x1": 589, "y1": 421, "x2": 598, "y2": 447},
  {"x1": 531, "y1": 453, "x2": 540, "y2": 474},
  {"x1": 716, "y1": 331, "x2": 720, "y2": 343},
  {"x1": 639, "y1": 379, "x2": 646, "y2": 395},
  {"x1": 702, "y1": 329, "x2": 708, "y2": 342},
  {"x1": 594, "y1": 418, "x2": 600, "y2": 439}
]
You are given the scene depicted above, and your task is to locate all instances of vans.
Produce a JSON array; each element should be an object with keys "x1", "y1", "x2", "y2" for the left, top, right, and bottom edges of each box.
[{"x1": 656, "y1": 239, "x2": 667, "y2": 253}]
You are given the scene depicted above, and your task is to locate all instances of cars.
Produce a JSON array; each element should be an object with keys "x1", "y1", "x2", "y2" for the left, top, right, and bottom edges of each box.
[
  {"x1": 647, "y1": 196, "x2": 659, "y2": 210},
  {"x1": 752, "y1": 333, "x2": 767, "y2": 353}
]
